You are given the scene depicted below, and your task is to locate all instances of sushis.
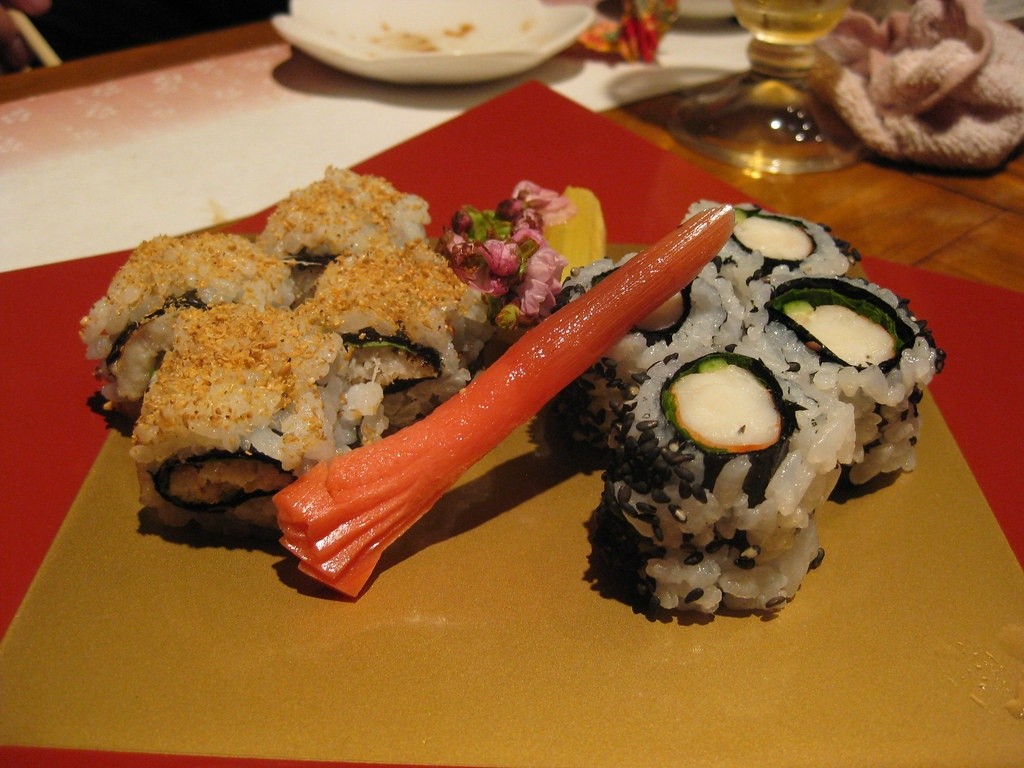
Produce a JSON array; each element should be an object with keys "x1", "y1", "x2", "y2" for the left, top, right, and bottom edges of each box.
[{"x1": 77, "y1": 163, "x2": 946, "y2": 615}]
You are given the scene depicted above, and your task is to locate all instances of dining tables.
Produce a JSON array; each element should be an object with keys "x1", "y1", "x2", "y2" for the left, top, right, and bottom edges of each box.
[{"x1": 2, "y1": 2, "x2": 1024, "y2": 768}]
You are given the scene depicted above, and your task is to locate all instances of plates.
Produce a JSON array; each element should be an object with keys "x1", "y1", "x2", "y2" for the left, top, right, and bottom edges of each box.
[{"x1": 270, "y1": 0, "x2": 595, "y2": 85}]
